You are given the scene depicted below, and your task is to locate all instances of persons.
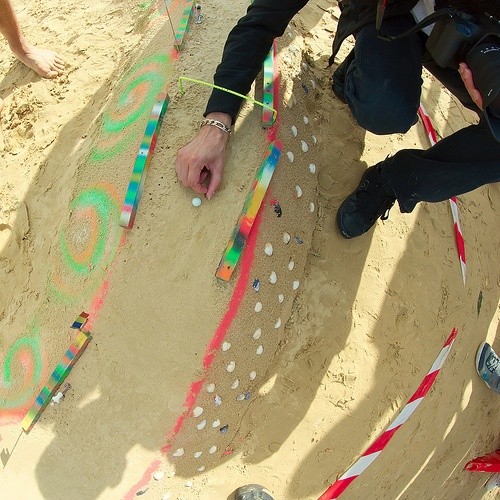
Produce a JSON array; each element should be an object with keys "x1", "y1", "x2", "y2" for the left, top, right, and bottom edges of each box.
[
  {"x1": 475, "y1": 341, "x2": 500, "y2": 393},
  {"x1": 1, "y1": 1, "x2": 67, "y2": 78},
  {"x1": 175, "y1": 1, "x2": 500, "y2": 241}
]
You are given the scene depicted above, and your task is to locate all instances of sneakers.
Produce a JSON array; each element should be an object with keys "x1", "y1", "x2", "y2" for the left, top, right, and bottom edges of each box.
[
  {"x1": 332, "y1": 46, "x2": 355, "y2": 101},
  {"x1": 476, "y1": 341, "x2": 500, "y2": 390},
  {"x1": 336, "y1": 161, "x2": 397, "y2": 239}
]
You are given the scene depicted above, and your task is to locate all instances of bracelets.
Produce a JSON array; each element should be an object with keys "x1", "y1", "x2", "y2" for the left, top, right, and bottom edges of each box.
[{"x1": 200, "y1": 119, "x2": 233, "y2": 136}]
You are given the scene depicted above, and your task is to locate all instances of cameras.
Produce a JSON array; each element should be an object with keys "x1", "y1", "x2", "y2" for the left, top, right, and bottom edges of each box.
[{"x1": 427, "y1": 1, "x2": 500, "y2": 141}]
